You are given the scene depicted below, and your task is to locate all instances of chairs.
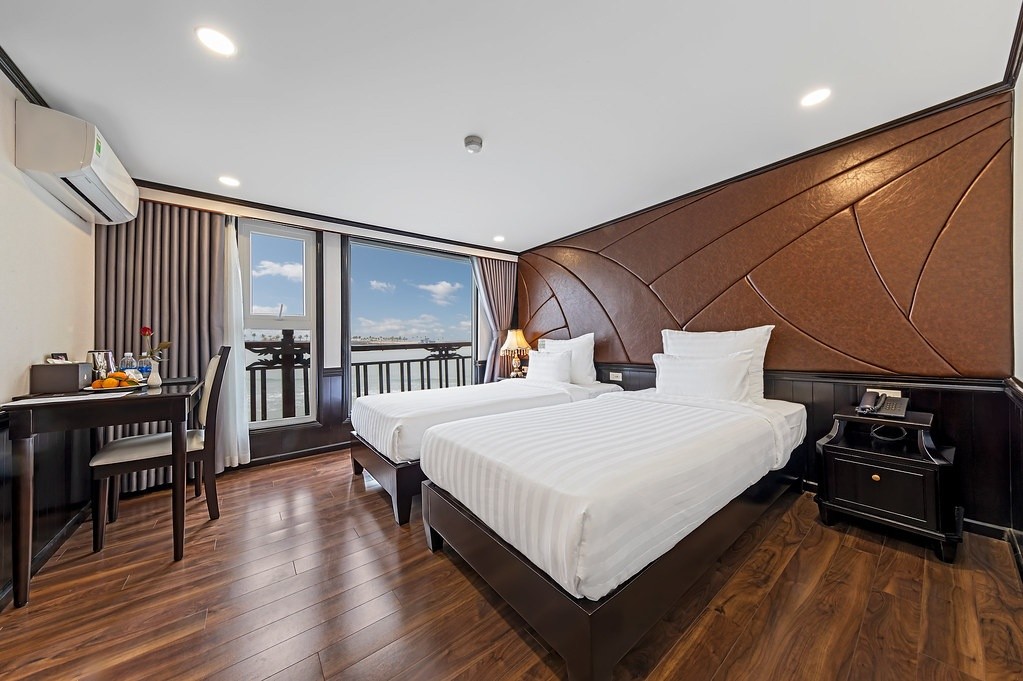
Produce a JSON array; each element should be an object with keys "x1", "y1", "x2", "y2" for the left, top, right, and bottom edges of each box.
[{"x1": 89, "y1": 345, "x2": 232, "y2": 553}]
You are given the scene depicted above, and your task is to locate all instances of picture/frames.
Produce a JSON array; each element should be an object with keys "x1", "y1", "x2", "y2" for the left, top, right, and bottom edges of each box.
[{"x1": 51, "y1": 353, "x2": 68, "y2": 361}]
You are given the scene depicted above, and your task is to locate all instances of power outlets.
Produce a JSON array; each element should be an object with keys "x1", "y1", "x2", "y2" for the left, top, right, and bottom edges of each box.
[
  {"x1": 521, "y1": 366, "x2": 528, "y2": 373},
  {"x1": 610, "y1": 372, "x2": 623, "y2": 381}
]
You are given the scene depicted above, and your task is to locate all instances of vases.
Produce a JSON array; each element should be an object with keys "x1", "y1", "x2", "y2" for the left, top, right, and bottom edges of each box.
[{"x1": 147, "y1": 360, "x2": 162, "y2": 388}]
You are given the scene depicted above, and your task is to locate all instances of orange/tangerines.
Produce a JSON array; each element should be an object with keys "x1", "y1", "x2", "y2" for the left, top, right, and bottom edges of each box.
[{"x1": 91, "y1": 371, "x2": 138, "y2": 387}]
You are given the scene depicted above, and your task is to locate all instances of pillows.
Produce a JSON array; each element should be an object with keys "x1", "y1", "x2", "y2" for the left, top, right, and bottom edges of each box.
[
  {"x1": 660, "y1": 324, "x2": 776, "y2": 406},
  {"x1": 538, "y1": 332, "x2": 597, "y2": 385},
  {"x1": 528, "y1": 350, "x2": 573, "y2": 383},
  {"x1": 652, "y1": 348, "x2": 754, "y2": 403}
]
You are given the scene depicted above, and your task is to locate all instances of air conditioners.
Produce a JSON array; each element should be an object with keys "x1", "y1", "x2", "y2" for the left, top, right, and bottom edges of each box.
[{"x1": 15, "y1": 99, "x2": 140, "y2": 225}]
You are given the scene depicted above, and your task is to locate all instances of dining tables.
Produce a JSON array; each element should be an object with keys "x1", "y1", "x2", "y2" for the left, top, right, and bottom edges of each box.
[{"x1": 0, "y1": 378, "x2": 206, "y2": 608}]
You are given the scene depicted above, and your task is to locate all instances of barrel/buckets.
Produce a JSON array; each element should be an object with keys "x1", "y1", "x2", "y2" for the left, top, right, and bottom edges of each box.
[{"x1": 87, "y1": 350, "x2": 113, "y2": 368}]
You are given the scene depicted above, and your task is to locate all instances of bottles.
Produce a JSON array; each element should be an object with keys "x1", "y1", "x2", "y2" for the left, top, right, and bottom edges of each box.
[
  {"x1": 119, "y1": 352, "x2": 137, "y2": 372},
  {"x1": 138, "y1": 352, "x2": 152, "y2": 378}
]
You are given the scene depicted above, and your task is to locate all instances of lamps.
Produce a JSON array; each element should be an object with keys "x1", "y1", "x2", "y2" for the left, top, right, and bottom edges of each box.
[{"x1": 500, "y1": 328, "x2": 532, "y2": 378}]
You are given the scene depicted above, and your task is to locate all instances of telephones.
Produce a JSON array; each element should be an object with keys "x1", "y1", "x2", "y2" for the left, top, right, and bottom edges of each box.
[{"x1": 857, "y1": 392, "x2": 910, "y2": 419}]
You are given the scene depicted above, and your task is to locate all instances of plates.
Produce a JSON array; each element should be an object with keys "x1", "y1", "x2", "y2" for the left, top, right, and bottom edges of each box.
[{"x1": 82, "y1": 383, "x2": 148, "y2": 391}]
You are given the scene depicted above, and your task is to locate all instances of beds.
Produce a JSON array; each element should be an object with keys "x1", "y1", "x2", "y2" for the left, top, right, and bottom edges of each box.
[
  {"x1": 348, "y1": 377, "x2": 625, "y2": 527},
  {"x1": 419, "y1": 388, "x2": 808, "y2": 681}
]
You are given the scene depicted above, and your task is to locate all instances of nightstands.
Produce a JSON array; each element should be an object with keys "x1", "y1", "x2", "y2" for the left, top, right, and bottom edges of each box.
[{"x1": 812, "y1": 405, "x2": 964, "y2": 564}]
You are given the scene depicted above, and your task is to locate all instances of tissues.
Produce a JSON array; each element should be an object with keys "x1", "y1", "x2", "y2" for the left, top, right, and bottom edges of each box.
[{"x1": 28, "y1": 357, "x2": 94, "y2": 392}]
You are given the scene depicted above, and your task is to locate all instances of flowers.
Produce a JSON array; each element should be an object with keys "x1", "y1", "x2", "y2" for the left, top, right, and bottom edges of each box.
[{"x1": 138, "y1": 326, "x2": 172, "y2": 358}]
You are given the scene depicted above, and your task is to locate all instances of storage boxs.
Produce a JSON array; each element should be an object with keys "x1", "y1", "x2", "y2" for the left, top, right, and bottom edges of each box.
[{"x1": 30, "y1": 362, "x2": 93, "y2": 394}]
